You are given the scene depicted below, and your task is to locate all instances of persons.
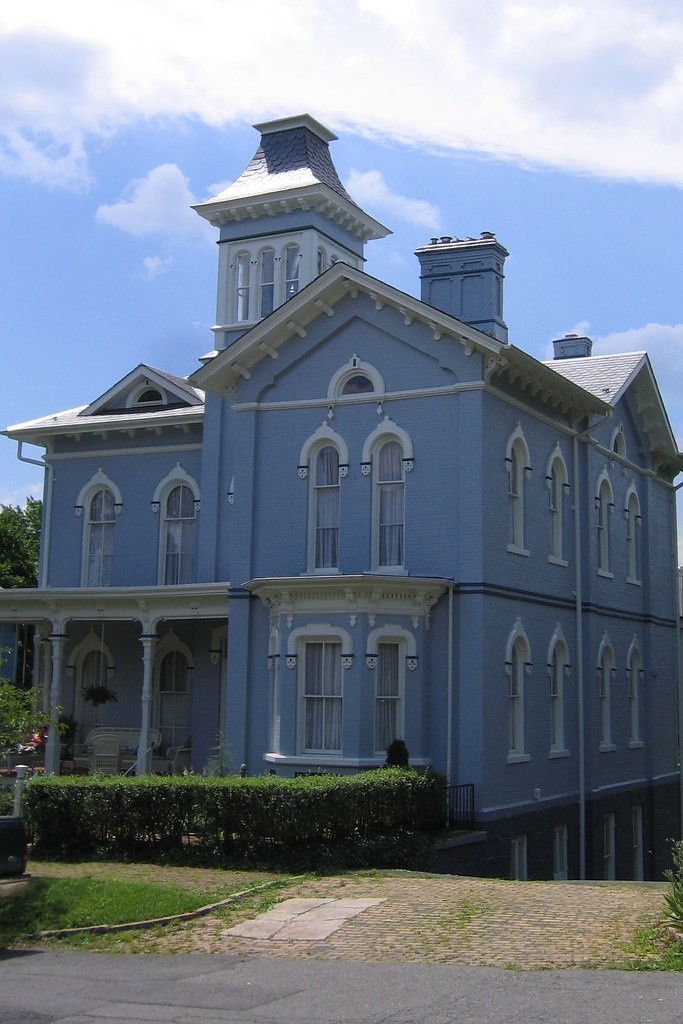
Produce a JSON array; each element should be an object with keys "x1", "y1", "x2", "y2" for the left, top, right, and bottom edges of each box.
[{"x1": 27, "y1": 725, "x2": 48, "y2": 749}]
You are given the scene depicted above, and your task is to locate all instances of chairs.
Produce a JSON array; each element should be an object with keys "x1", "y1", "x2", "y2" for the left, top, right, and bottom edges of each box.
[
  {"x1": 167, "y1": 746, "x2": 192, "y2": 772},
  {"x1": 87, "y1": 735, "x2": 123, "y2": 776}
]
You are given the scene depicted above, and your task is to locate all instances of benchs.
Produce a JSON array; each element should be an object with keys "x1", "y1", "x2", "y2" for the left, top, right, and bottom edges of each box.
[{"x1": 73, "y1": 727, "x2": 162, "y2": 775}]
